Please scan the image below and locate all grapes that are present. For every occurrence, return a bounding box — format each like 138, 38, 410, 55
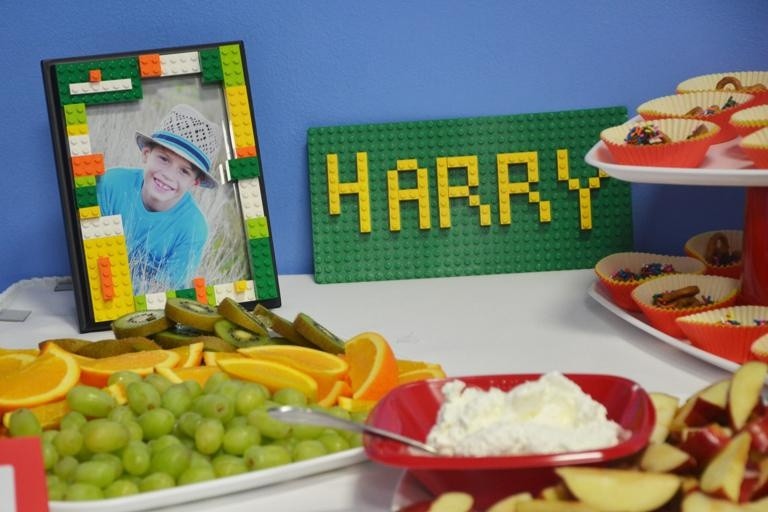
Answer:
8, 371, 368, 500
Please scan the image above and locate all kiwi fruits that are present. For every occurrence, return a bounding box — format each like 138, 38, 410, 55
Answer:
39, 296, 346, 359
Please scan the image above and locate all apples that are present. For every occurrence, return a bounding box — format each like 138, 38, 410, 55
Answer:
396, 360, 768, 512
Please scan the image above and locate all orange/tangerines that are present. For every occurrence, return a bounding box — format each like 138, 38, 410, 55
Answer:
0, 332, 446, 432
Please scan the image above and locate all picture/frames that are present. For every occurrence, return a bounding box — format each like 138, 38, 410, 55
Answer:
38, 37, 281, 332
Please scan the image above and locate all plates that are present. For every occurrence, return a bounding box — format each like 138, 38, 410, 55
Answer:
43, 448, 378, 511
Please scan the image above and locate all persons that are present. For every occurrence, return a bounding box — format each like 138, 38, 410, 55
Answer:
93, 99, 223, 297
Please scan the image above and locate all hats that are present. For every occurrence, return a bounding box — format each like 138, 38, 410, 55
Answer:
135, 104, 224, 191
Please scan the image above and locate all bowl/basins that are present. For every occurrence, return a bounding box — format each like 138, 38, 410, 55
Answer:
361, 376, 655, 502
